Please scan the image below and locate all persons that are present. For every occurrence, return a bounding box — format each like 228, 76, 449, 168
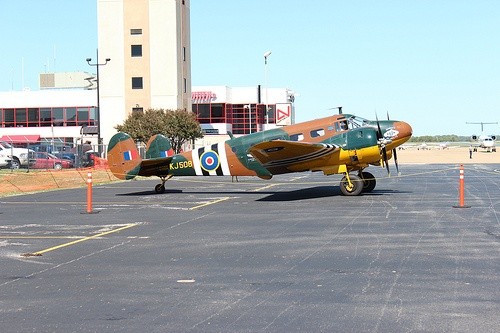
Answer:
468, 144, 473, 159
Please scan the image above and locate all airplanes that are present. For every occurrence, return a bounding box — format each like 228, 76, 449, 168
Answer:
466, 121, 500, 153
398, 142, 460, 152
106, 105, 412, 196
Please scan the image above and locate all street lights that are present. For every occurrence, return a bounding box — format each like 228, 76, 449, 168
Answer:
86, 48, 111, 157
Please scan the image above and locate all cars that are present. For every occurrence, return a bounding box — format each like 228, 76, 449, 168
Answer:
53, 152, 100, 168
29, 152, 72, 170
0, 146, 13, 169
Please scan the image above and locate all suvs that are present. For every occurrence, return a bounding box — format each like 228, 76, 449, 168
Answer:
0, 140, 36, 169
